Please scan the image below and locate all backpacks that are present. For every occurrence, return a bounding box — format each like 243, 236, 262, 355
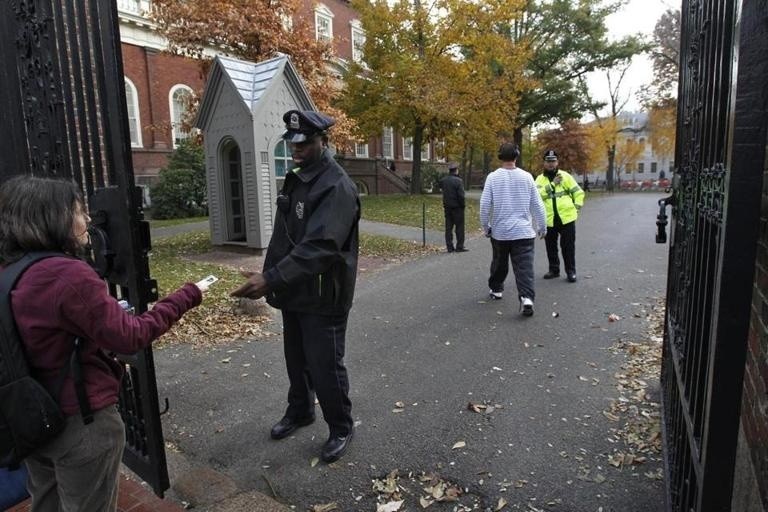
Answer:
0, 250, 94, 466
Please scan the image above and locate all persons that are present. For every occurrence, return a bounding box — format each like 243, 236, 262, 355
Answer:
227, 109, 362, 462
441, 160, 468, 253
534, 150, 586, 282
389, 159, 395, 175
478, 142, 548, 318
0, 173, 210, 511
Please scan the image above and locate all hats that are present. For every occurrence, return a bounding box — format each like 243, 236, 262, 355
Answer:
282, 110, 334, 143
543, 150, 558, 162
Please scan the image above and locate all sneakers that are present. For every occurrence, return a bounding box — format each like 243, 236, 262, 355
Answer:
489, 289, 502, 300
521, 296, 533, 316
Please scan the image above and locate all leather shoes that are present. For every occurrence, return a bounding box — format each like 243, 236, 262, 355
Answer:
271, 412, 316, 439
544, 272, 560, 278
322, 433, 352, 461
568, 272, 576, 282
456, 247, 469, 252
